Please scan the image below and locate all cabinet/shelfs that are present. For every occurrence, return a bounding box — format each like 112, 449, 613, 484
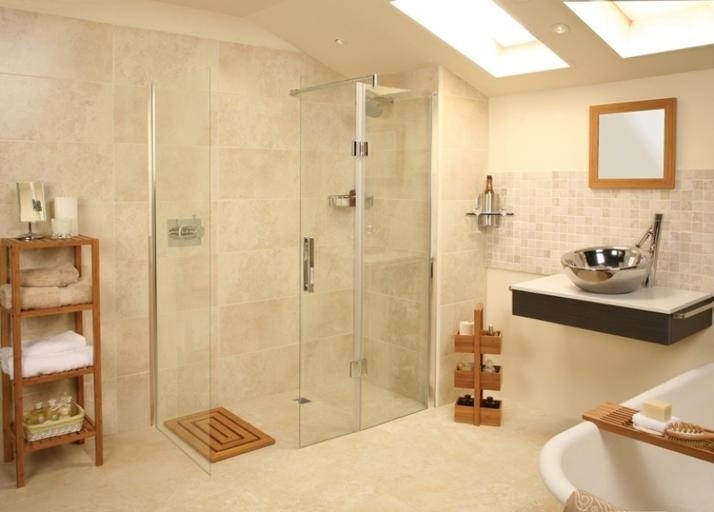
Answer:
2, 230, 104, 486
453, 304, 503, 428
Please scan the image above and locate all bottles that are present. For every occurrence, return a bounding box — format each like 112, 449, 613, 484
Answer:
29, 398, 60, 425
485, 175, 495, 194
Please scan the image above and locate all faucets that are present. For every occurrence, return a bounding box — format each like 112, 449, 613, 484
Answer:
635, 213, 663, 288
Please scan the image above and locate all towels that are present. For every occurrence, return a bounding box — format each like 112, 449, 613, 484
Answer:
2, 260, 95, 311
632, 413, 679, 437
1, 330, 94, 381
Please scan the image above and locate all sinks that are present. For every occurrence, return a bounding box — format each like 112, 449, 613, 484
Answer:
561, 246, 651, 294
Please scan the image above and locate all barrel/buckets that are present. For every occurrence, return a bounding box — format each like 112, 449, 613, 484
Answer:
479, 192, 501, 227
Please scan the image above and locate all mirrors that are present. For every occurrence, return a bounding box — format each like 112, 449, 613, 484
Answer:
587, 97, 678, 191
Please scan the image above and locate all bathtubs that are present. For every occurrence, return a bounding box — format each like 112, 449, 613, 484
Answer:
540, 362, 714, 512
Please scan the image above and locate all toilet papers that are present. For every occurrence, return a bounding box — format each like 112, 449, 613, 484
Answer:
460, 321, 474, 336
53, 196, 78, 235
